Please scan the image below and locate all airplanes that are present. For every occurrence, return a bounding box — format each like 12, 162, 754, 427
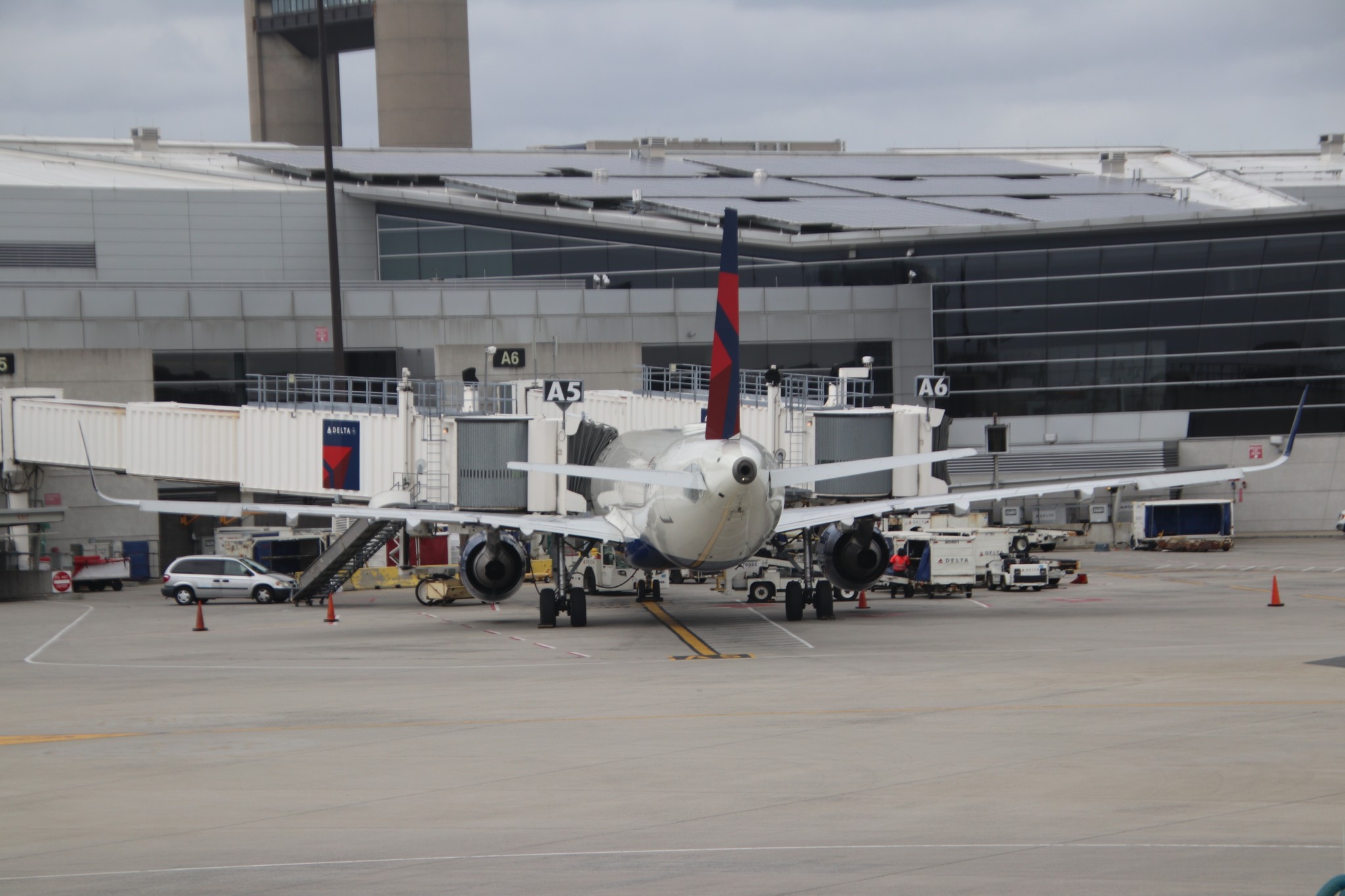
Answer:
94, 207, 1313, 628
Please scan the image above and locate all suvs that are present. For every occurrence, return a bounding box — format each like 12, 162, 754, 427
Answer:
161, 554, 300, 605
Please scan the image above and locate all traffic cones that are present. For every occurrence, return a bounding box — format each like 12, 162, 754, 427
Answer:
322, 593, 339, 622
190, 599, 209, 632
1266, 573, 1291, 610
856, 590, 874, 610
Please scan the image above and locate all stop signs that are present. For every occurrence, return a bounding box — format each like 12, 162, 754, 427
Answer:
51, 571, 74, 594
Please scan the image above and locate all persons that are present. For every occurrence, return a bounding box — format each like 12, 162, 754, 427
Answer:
887, 548, 914, 599
